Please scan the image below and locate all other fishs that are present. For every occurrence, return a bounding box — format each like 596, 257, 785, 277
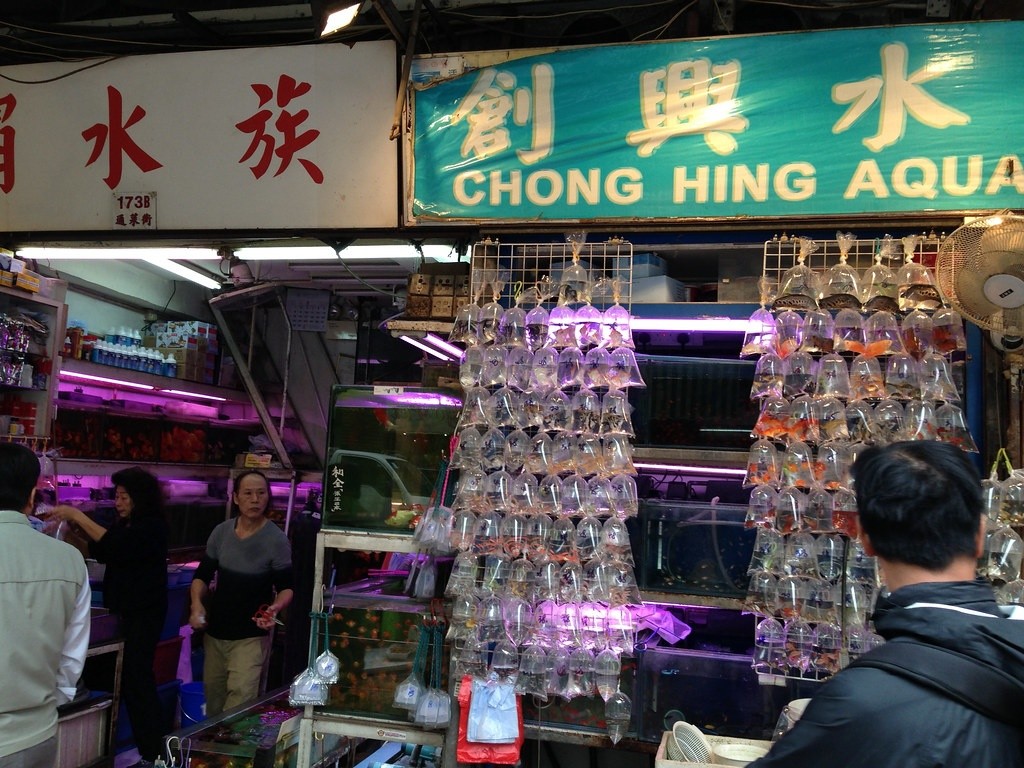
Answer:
190, 726, 255, 768
738, 264, 1024, 683
443, 299, 639, 704
318, 610, 450, 712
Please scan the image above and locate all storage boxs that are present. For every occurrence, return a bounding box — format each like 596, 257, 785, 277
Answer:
521, 655, 638, 729
655, 730, 773, 768
145, 319, 218, 383
208, 425, 251, 465
106, 410, 161, 462
161, 682, 300, 768
53, 401, 106, 460
320, 596, 449, 721
321, 382, 464, 534
645, 499, 756, 597
163, 415, 209, 464
58, 699, 113, 768
639, 646, 762, 743
0, 243, 67, 302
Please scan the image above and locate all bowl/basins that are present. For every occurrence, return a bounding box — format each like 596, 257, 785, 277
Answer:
710, 739, 771, 768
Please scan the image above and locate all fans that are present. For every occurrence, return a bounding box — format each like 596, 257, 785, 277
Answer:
935, 215, 1024, 353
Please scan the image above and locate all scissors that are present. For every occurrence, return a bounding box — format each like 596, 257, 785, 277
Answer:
255, 603, 285, 626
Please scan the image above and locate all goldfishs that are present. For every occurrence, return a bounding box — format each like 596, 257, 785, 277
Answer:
54, 419, 228, 463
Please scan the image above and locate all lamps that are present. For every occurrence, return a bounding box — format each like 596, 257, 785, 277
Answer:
15, 237, 455, 289
391, 329, 464, 362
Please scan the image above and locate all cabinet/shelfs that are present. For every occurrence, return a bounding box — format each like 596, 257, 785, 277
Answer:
97, 498, 225, 556
294, 443, 761, 768
0, 285, 70, 448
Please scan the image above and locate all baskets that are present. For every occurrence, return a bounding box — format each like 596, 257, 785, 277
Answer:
667, 720, 716, 764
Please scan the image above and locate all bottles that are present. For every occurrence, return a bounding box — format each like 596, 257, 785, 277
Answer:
105, 327, 142, 347
90, 338, 180, 378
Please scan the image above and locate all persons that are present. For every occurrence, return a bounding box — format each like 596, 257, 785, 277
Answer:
744, 439, 1024, 768
0, 441, 91, 768
46, 468, 167, 768
190, 472, 322, 719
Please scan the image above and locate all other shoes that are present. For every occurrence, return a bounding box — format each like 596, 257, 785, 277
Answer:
125, 759, 154, 768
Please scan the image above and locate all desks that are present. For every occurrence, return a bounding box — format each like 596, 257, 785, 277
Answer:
70, 639, 126, 768
